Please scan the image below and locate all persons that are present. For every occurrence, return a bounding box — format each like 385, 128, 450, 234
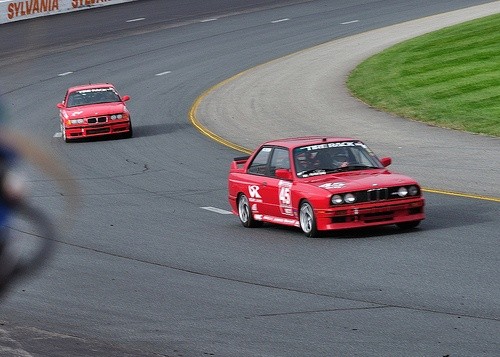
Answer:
329, 153, 349, 168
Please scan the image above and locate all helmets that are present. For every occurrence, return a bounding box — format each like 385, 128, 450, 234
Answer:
332, 147, 349, 166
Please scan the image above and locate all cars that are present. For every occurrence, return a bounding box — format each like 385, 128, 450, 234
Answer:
56, 82, 132, 141
226, 135, 425, 237
